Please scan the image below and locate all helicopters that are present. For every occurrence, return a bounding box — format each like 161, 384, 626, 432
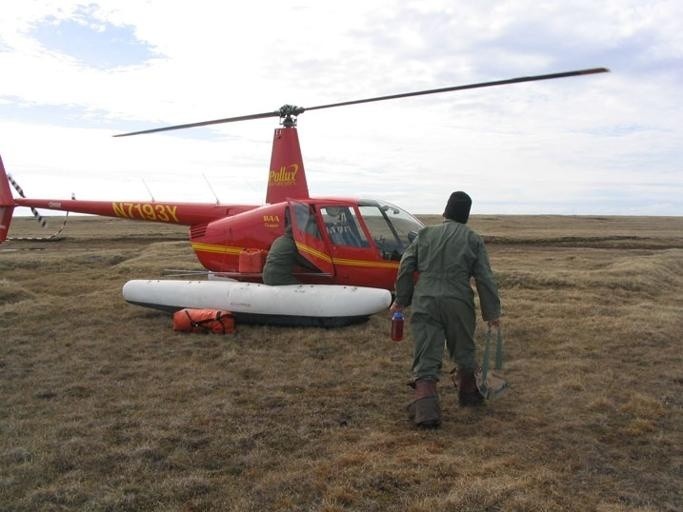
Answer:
0, 65, 610, 326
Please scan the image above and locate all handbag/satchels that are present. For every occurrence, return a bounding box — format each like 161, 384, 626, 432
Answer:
477, 368, 508, 399
173, 308, 234, 333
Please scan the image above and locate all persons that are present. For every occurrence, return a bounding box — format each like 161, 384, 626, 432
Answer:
263, 223, 322, 285
390, 192, 501, 426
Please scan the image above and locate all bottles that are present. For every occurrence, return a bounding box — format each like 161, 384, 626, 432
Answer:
390, 311, 403, 342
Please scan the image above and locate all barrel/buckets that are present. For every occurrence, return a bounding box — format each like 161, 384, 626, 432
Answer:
239, 247, 265, 274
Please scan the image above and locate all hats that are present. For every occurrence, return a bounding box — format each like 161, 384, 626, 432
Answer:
442, 191, 471, 223
